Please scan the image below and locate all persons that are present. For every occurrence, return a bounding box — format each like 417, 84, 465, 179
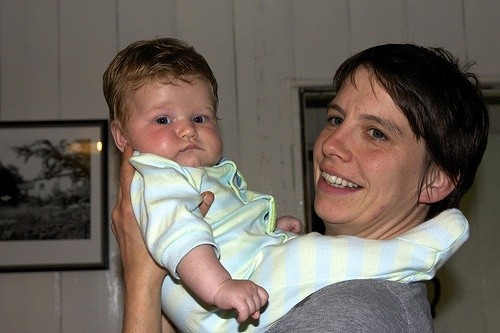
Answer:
117, 41, 491, 331
103, 31, 306, 333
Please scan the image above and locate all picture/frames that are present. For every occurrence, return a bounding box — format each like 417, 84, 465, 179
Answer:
1, 118, 109, 274
